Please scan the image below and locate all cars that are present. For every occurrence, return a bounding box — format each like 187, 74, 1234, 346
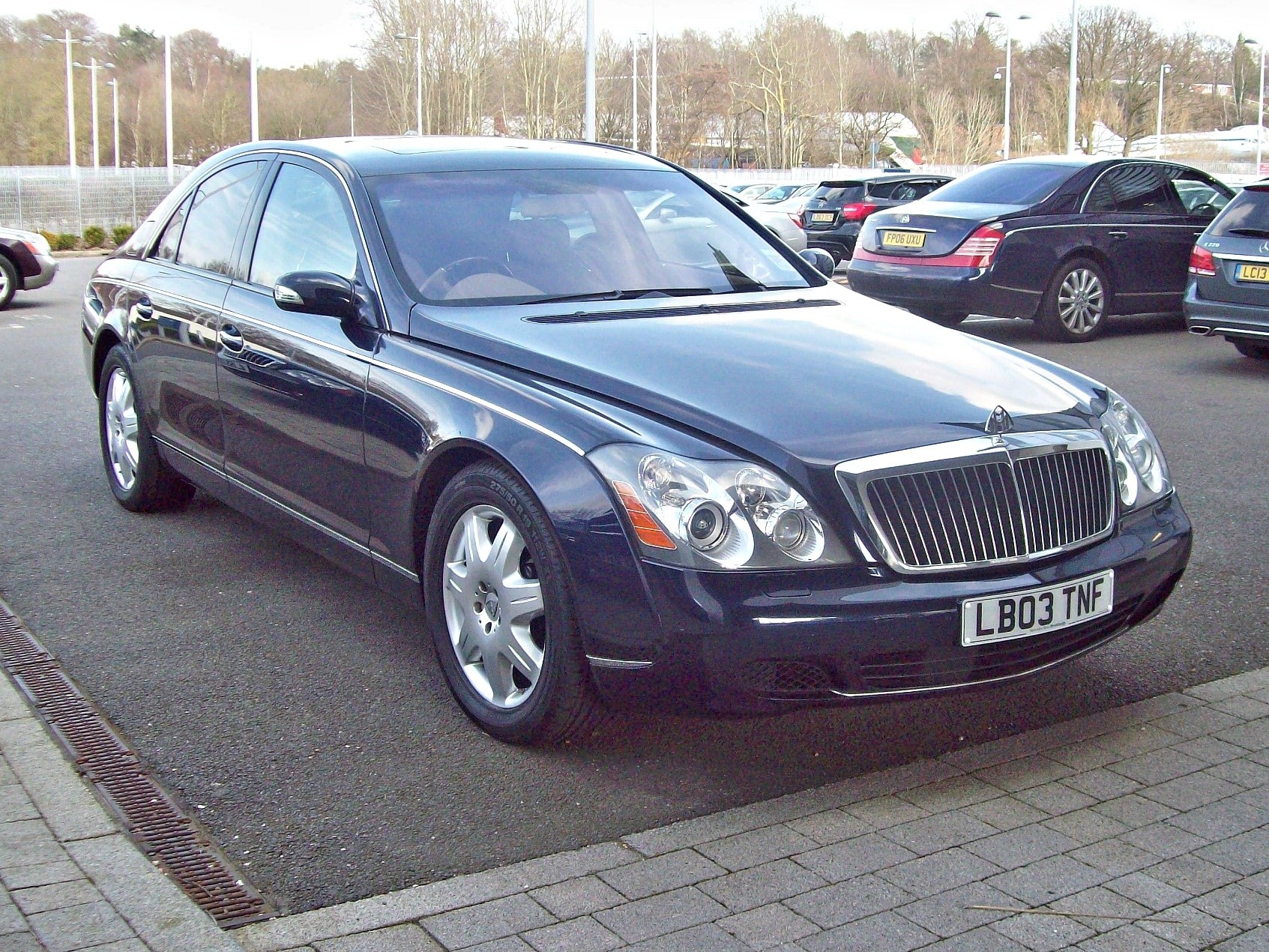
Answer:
80, 131, 1194, 750
570, 173, 819, 257
0, 226, 60, 311
845, 157, 1269, 360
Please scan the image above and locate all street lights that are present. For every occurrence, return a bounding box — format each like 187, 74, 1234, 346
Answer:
1156, 63, 1172, 158
629, 30, 649, 149
1243, 38, 1266, 182
394, 26, 423, 138
985, 9, 1032, 160
42, 28, 121, 179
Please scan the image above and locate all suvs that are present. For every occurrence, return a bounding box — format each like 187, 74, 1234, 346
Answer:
802, 169, 958, 266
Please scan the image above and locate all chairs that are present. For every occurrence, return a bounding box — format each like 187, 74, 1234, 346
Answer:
499, 219, 614, 295
912, 187, 930, 200
1140, 201, 1167, 213
